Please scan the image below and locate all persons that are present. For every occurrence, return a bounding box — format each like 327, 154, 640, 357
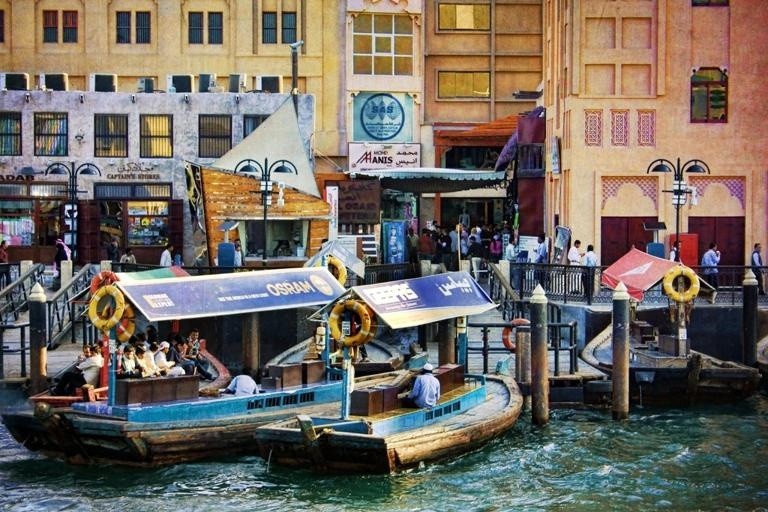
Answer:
568, 240, 585, 294
494, 223, 500, 235
429, 220, 439, 232
670, 240, 683, 265
581, 245, 598, 304
459, 207, 471, 229
479, 147, 500, 170
701, 242, 722, 292
107, 237, 122, 273
401, 363, 441, 408
234, 244, 243, 269
222, 369, 258, 398
490, 236, 502, 262
0, 239, 12, 288
501, 224, 510, 233
480, 224, 492, 261
464, 236, 483, 283
467, 228, 483, 245
450, 223, 463, 270
513, 203, 520, 246
407, 228, 419, 260
431, 232, 440, 263
159, 243, 175, 267
488, 223, 494, 232
461, 230, 470, 259
233, 237, 248, 267
120, 247, 137, 270
50, 325, 220, 395
54, 238, 74, 274
438, 228, 451, 270
751, 241, 766, 296
417, 229, 434, 260
405, 230, 412, 260
506, 236, 521, 289
533, 232, 549, 292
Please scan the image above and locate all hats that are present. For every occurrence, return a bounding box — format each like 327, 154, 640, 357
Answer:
423, 363, 434, 371
157, 341, 170, 350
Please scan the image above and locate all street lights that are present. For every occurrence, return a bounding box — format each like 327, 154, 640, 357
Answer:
647, 156, 709, 259
235, 156, 297, 260
45, 163, 101, 266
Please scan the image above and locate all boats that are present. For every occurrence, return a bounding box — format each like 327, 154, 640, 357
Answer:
254, 271, 522, 474
1, 266, 196, 454
581, 246, 762, 412
4, 271, 423, 463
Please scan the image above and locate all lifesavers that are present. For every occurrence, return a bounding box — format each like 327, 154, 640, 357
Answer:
314, 255, 348, 286
502, 319, 532, 354
102, 301, 136, 343
663, 265, 700, 303
330, 299, 372, 347
88, 284, 126, 329
337, 301, 377, 348
91, 271, 119, 296
115, 311, 128, 336
